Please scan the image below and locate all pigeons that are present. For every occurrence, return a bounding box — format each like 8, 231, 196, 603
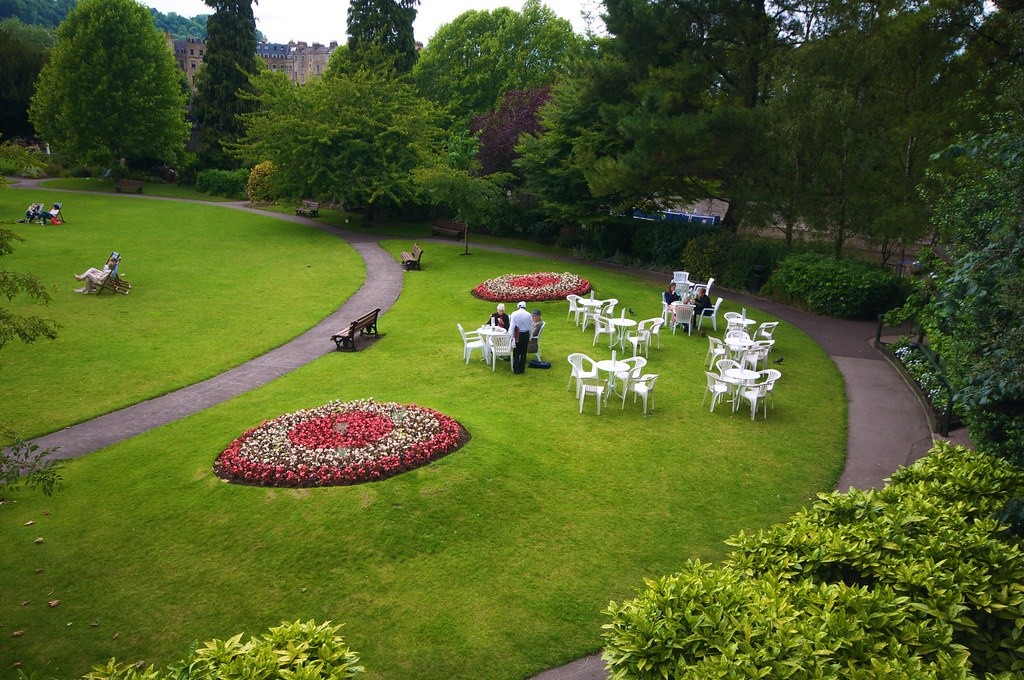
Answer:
773, 357, 783, 364
629, 308, 634, 314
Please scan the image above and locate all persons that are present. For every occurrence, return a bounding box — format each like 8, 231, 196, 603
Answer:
46, 143, 50, 155
508, 301, 533, 376
692, 287, 713, 328
26, 204, 40, 223
75, 257, 116, 295
665, 283, 681, 307
487, 303, 509, 331
504, 310, 542, 361
38, 204, 60, 226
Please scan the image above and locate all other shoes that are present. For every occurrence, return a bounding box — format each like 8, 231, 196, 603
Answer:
74, 273, 81, 282
82, 292, 90, 295
504, 357, 511, 361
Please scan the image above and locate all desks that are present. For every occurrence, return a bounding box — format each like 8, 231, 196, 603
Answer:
725, 369, 760, 412
729, 318, 757, 333
477, 326, 507, 362
724, 337, 754, 363
608, 318, 637, 355
595, 360, 630, 406
578, 299, 603, 329
674, 281, 695, 287
669, 301, 690, 331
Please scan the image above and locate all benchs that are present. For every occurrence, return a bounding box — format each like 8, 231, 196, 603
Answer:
431, 218, 465, 241
400, 243, 424, 271
115, 179, 145, 194
331, 308, 381, 352
296, 200, 319, 219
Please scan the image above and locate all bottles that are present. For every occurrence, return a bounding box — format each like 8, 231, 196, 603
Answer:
492, 323, 495, 333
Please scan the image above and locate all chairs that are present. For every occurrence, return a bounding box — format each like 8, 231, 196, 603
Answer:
25, 203, 44, 223
456, 271, 779, 421
49, 202, 66, 222
95, 251, 132, 295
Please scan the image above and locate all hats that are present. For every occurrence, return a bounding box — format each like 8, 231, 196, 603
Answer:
533, 310, 541, 315
517, 301, 526, 308
497, 304, 505, 310
